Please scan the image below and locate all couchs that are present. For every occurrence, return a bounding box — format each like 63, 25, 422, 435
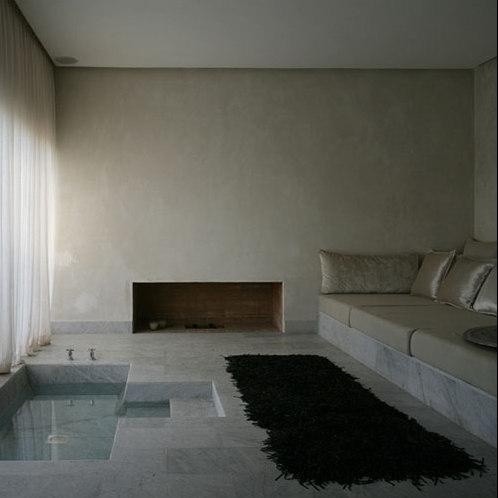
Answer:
316, 293, 497, 449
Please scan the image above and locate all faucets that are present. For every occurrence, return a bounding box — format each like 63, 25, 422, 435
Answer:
66, 349, 74, 360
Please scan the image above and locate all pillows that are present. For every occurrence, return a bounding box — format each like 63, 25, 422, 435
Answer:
319, 249, 419, 295
433, 254, 495, 312
471, 265, 497, 317
410, 249, 456, 303
462, 237, 497, 260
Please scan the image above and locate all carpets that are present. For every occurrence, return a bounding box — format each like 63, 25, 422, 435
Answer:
223, 354, 487, 493
